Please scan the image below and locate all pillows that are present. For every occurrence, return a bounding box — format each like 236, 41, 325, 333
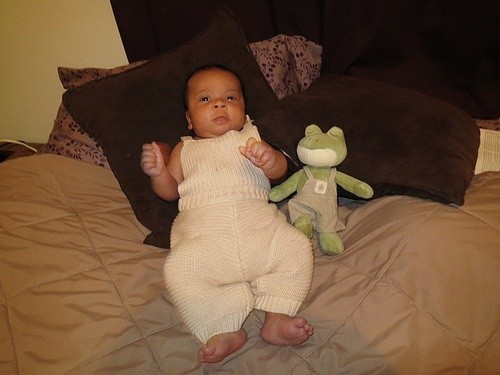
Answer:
30, 58, 148, 170
249, 35, 323, 99
62, 12, 284, 247
256, 67, 482, 205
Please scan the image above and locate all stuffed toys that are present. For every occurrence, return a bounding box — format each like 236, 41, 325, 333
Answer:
268, 125, 375, 256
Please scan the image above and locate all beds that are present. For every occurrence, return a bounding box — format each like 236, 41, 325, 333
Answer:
1, 142, 500, 375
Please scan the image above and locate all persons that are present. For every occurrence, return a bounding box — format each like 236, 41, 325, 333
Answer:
142, 64, 316, 364
